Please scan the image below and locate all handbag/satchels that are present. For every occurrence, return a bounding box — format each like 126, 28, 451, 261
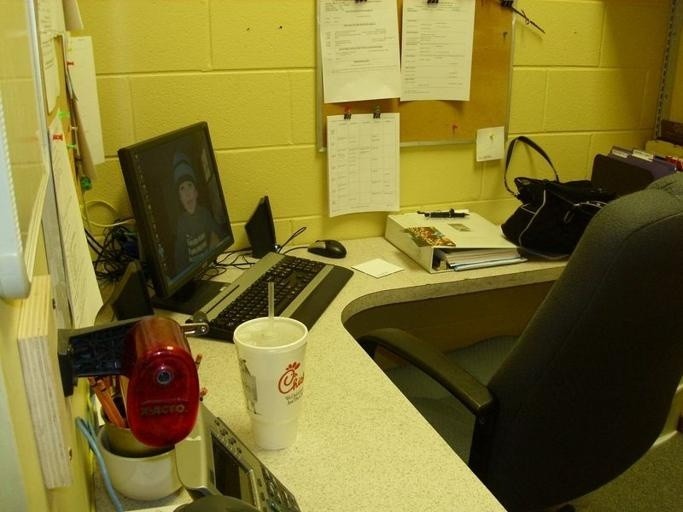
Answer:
501, 136, 623, 261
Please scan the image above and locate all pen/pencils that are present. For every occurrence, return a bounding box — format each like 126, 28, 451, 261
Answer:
90, 374, 127, 430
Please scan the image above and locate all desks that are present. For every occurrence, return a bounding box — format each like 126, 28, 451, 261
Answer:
92, 235, 569, 512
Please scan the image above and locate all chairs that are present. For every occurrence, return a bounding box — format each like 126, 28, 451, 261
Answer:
357, 172, 683, 512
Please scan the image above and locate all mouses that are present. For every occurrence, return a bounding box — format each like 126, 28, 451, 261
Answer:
307, 239, 346, 258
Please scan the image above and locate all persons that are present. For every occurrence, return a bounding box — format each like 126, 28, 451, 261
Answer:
172, 152, 222, 275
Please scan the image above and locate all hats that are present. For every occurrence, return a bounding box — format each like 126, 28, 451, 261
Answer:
172, 152, 198, 197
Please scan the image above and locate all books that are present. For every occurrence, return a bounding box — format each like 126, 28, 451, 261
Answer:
384, 209, 529, 274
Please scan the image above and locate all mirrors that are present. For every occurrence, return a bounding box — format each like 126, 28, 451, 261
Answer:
0, 0, 49, 299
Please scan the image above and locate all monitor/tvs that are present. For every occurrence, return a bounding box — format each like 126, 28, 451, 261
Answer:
116, 121, 233, 315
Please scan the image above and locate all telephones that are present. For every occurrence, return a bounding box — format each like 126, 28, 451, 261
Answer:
172, 396, 300, 512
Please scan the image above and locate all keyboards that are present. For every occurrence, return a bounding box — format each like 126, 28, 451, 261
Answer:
192, 251, 355, 345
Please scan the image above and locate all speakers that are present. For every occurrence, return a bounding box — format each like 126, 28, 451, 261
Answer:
244, 196, 277, 258
109, 261, 154, 321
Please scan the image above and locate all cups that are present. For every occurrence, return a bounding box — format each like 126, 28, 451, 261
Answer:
89, 428, 186, 501
100, 393, 170, 454
234, 315, 309, 451
